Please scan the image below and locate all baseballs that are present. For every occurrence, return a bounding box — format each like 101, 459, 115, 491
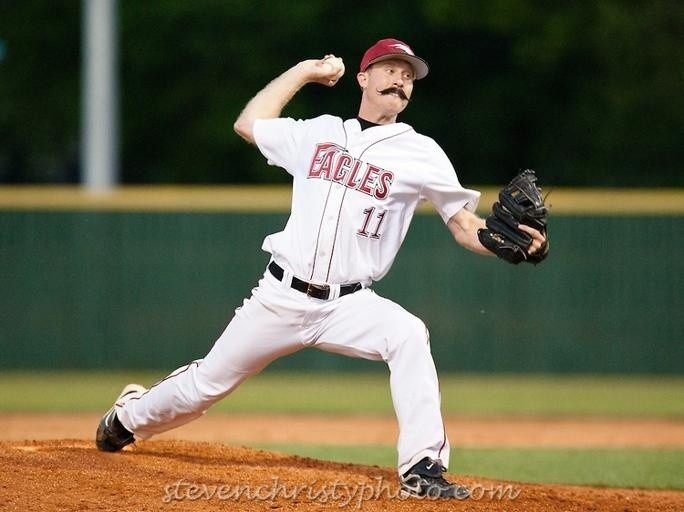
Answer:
323, 56, 344, 80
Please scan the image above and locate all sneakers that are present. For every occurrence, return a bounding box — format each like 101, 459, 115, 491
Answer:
97, 384, 147, 452
399, 460, 470, 501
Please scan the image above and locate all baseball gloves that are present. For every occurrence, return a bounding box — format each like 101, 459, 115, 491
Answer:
476, 168, 549, 265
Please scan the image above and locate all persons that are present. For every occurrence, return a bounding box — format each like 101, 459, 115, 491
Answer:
95, 35, 548, 501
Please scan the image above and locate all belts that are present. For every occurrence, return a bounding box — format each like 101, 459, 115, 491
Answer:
267, 261, 362, 300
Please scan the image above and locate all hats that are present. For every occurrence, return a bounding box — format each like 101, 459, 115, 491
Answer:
360, 38, 429, 81
